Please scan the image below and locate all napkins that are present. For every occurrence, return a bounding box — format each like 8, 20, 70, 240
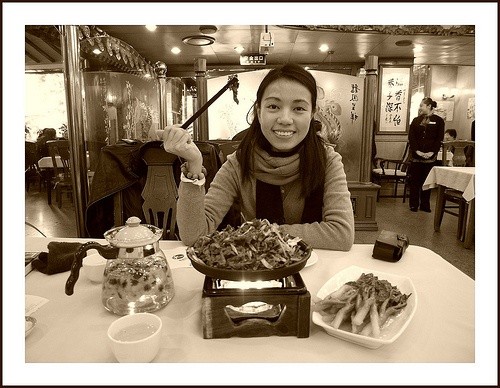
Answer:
31, 240, 87, 275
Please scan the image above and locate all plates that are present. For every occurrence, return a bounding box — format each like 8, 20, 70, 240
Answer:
185, 232, 312, 282
25, 316, 38, 339
312, 266, 418, 352
304, 251, 319, 268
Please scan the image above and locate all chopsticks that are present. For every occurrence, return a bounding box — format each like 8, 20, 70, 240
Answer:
180, 77, 237, 129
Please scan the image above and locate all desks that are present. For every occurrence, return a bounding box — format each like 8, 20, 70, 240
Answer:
38, 156, 72, 204
24, 235, 475, 363
422, 166, 475, 250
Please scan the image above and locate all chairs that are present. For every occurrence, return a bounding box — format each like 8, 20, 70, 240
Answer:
100, 141, 219, 242
440, 141, 475, 240
371, 141, 410, 203
25, 138, 75, 209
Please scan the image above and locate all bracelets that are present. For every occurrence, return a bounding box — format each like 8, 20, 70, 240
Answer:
180, 161, 208, 181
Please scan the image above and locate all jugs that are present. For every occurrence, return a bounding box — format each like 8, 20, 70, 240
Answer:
65, 216, 176, 317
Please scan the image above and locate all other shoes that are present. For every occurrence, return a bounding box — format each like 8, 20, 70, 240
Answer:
420, 207, 431, 212
410, 206, 418, 212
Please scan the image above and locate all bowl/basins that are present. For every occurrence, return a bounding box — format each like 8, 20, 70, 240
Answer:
80, 252, 108, 283
106, 312, 163, 363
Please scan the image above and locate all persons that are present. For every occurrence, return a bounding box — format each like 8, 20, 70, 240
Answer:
445, 128, 457, 140
408, 96, 445, 212
155, 63, 354, 252
